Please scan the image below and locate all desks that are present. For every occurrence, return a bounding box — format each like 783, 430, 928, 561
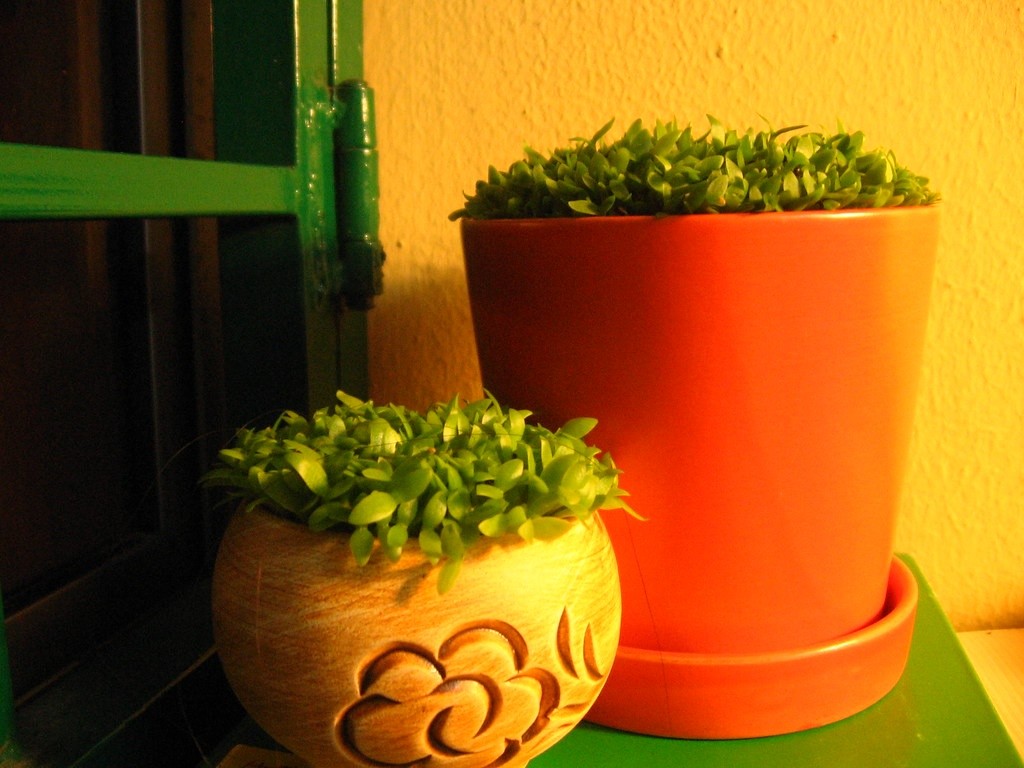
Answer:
195, 552, 1024, 768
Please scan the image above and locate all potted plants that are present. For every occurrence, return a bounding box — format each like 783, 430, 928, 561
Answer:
447, 113, 944, 740
218, 389, 646, 768
